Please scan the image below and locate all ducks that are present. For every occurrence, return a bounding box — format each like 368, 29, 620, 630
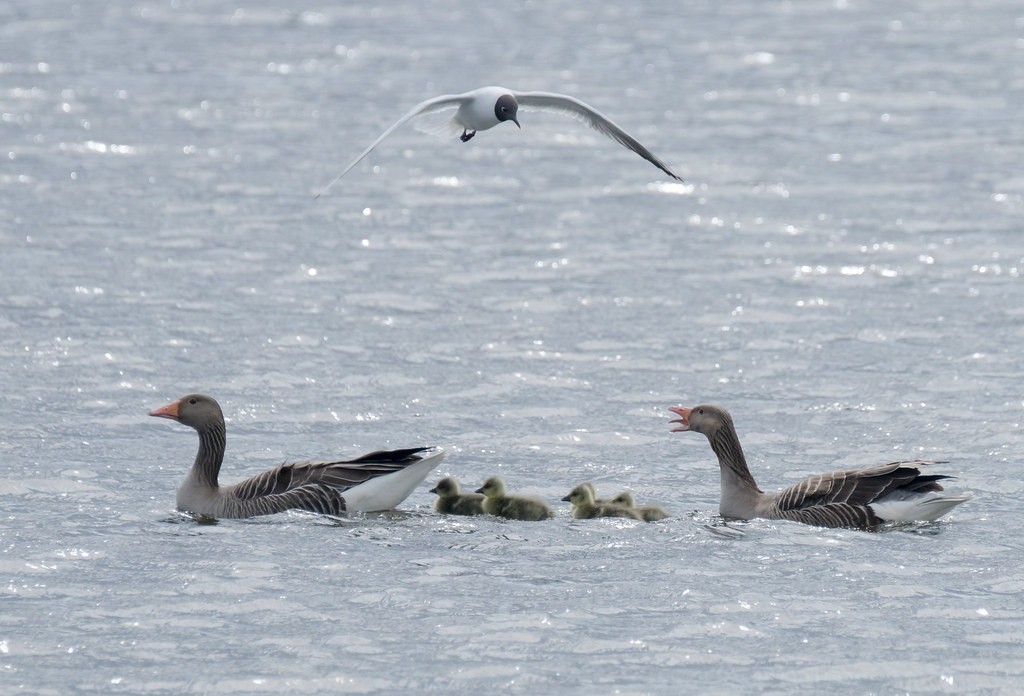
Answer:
668, 405, 969, 534
428, 478, 485, 516
561, 483, 672, 523
148, 394, 448, 520
475, 476, 557, 521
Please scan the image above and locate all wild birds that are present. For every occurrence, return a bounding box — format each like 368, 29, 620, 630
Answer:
314, 86, 685, 198
